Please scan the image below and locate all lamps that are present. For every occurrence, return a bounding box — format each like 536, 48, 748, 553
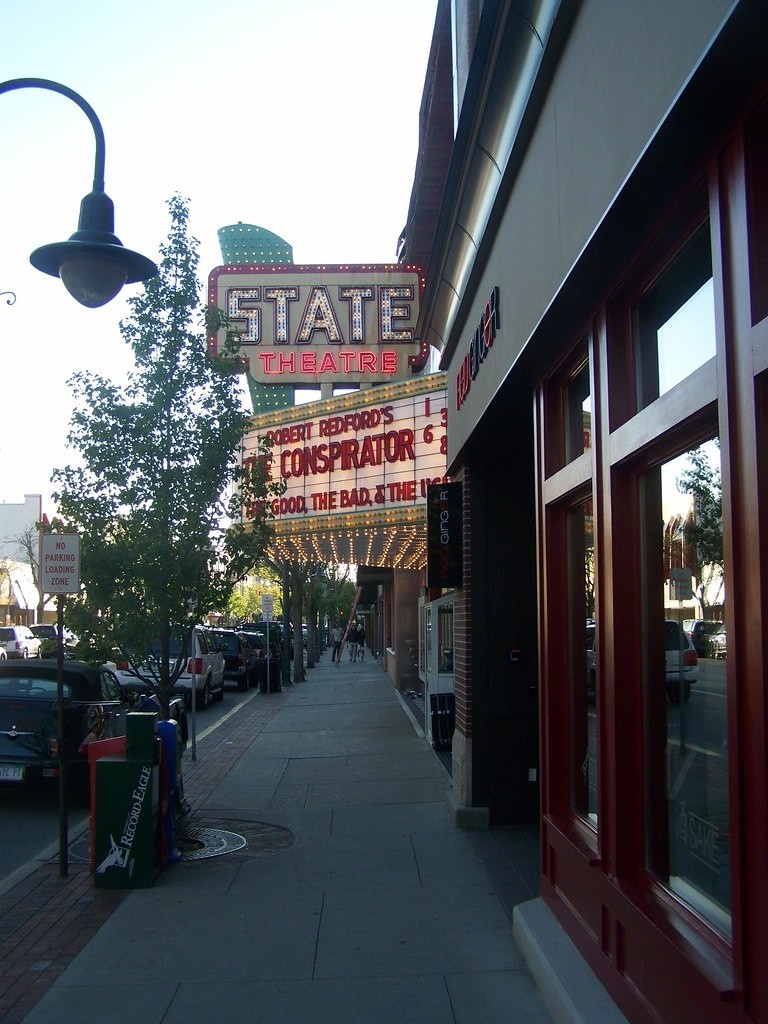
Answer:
1, 76, 159, 312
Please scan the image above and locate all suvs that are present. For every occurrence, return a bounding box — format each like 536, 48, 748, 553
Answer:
95, 622, 225, 711
580, 618, 699, 703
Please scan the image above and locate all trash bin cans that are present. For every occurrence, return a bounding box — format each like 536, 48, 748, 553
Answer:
258, 655, 283, 694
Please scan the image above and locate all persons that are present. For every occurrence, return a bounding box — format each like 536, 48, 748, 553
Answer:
357, 624, 365, 663
330, 623, 345, 662
343, 624, 360, 662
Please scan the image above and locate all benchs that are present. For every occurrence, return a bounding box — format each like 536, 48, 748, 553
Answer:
0, 687, 67, 701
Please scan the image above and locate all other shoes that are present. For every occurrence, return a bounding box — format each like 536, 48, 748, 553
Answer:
353, 660, 356, 663
331, 660, 335, 663
350, 659, 352, 662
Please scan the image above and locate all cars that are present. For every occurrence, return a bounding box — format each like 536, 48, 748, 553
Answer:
28, 623, 72, 660
0, 658, 143, 811
682, 619, 728, 659
202, 619, 330, 693
1, 622, 43, 660
